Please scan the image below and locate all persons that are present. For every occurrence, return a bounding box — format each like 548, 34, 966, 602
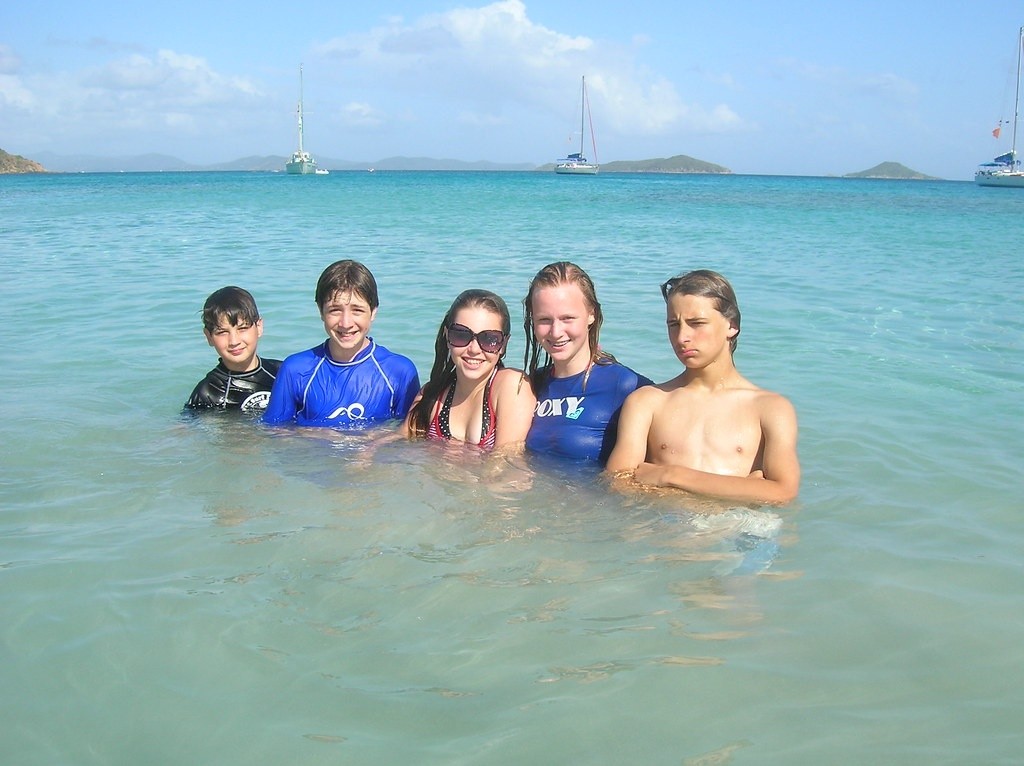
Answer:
606, 269, 800, 515
181, 286, 284, 421
518, 262, 654, 480
404, 290, 538, 455
260, 259, 421, 434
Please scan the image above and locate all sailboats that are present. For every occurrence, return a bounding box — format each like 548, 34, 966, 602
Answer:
556, 76, 599, 175
974, 26, 1023, 187
286, 62, 318, 178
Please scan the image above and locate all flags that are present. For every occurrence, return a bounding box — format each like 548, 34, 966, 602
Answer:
993, 127, 1000, 138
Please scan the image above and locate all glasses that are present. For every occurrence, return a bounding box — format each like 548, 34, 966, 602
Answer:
447, 322, 506, 354
